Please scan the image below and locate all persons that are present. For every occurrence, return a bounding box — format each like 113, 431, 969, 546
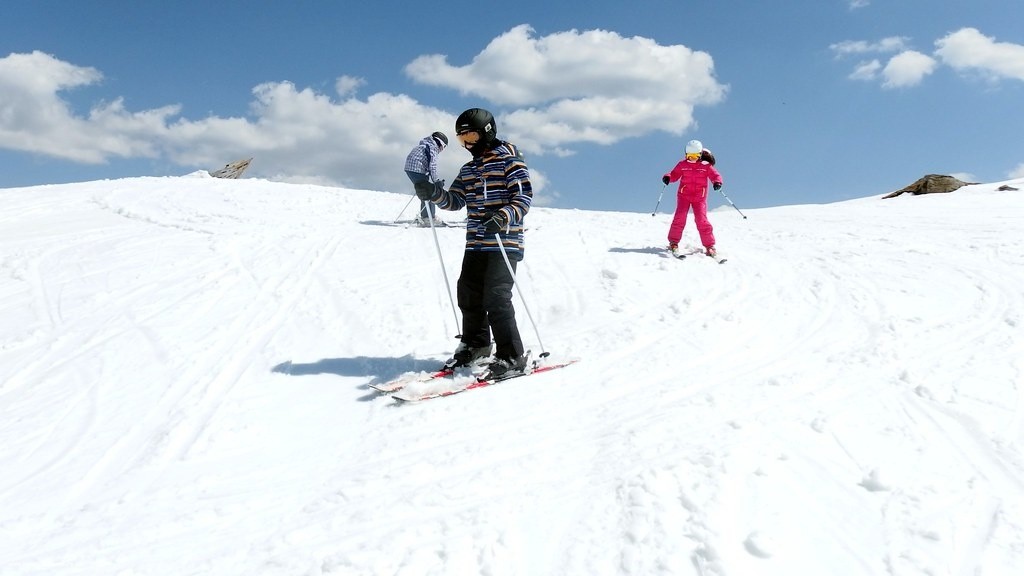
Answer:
404, 132, 448, 226
415, 108, 532, 377
663, 140, 723, 254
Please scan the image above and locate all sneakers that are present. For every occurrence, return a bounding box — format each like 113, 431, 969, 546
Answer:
477, 352, 528, 385
423, 216, 445, 228
440, 341, 493, 374
706, 246, 716, 256
669, 242, 681, 253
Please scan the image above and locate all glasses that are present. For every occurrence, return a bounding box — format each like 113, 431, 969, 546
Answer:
456, 129, 483, 147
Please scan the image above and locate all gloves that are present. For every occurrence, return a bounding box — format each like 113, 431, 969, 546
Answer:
713, 182, 721, 191
662, 176, 670, 186
416, 181, 440, 201
480, 213, 506, 239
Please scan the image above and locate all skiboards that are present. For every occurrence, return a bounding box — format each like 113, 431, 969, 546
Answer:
394, 218, 469, 228
366, 356, 581, 405
668, 239, 728, 266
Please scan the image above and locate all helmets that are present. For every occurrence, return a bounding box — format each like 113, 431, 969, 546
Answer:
455, 108, 497, 152
685, 139, 703, 160
431, 131, 448, 150
